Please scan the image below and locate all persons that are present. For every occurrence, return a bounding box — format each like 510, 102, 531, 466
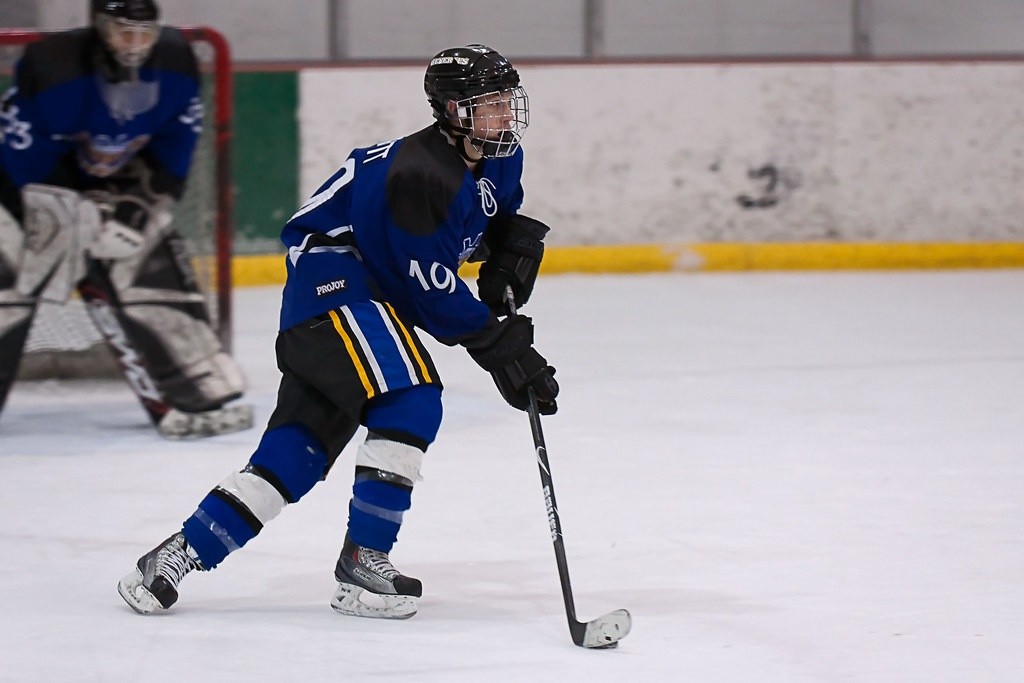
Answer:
0, 0, 206, 416
115, 44, 559, 619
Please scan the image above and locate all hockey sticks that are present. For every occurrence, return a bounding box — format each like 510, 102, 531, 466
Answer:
63, 250, 255, 438
500, 285, 632, 647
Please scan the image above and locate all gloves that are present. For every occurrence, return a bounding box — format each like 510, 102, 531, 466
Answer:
467, 213, 551, 318
464, 314, 559, 415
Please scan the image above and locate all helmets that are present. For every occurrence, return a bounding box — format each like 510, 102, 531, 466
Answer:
424, 43, 530, 159
89, 0, 160, 69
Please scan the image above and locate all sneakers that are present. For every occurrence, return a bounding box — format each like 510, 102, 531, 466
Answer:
331, 534, 422, 620
116, 531, 211, 615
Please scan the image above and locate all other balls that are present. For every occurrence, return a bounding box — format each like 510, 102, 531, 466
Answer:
593, 642, 619, 650
